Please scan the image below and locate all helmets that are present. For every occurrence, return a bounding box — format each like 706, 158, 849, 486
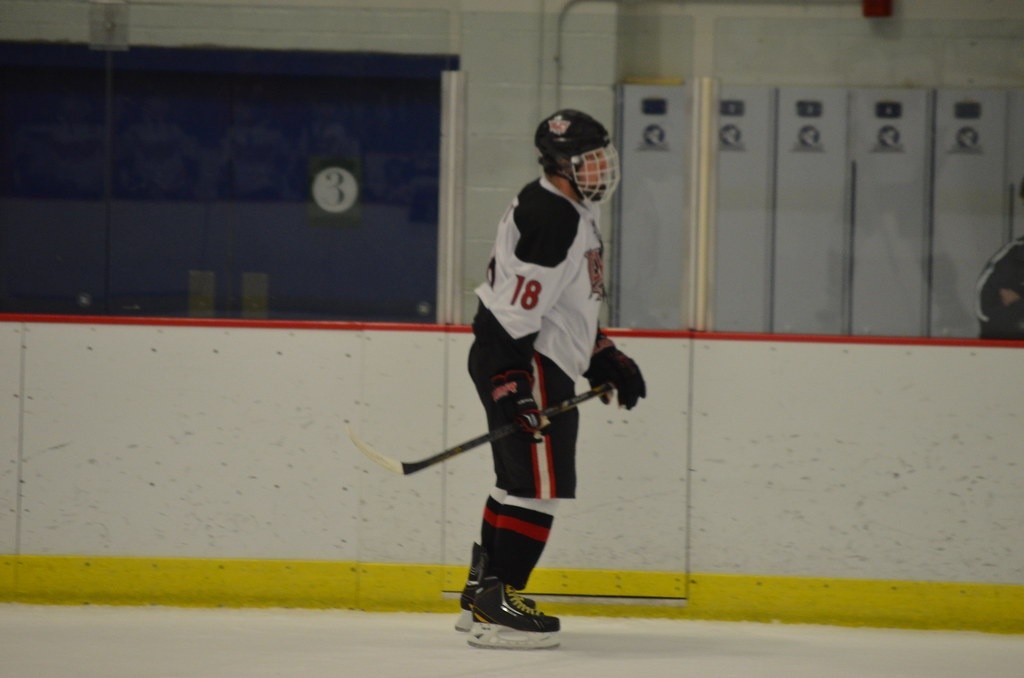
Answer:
534, 109, 620, 205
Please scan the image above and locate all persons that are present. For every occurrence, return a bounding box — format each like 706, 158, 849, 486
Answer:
972, 235, 1024, 341
455, 109, 649, 647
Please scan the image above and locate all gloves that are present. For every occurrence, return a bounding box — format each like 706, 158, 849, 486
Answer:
582, 334, 646, 410
489, 369, 552, 443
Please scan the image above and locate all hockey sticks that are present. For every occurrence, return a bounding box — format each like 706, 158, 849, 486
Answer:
347, 380, 615, 477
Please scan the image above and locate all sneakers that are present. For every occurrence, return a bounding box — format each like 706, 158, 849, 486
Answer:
455, 541, 537, 632
467, 553, 562, 647
